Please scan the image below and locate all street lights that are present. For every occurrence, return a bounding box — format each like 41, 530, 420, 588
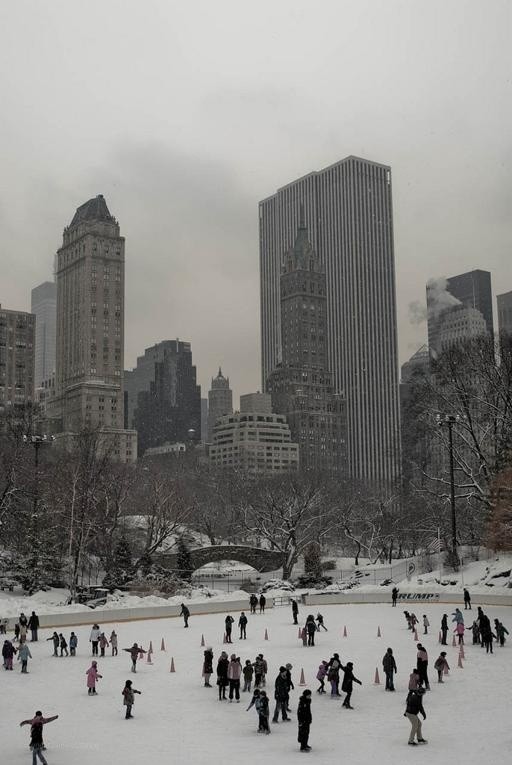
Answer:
434, 412, 469, 574
22, 433, 56, 562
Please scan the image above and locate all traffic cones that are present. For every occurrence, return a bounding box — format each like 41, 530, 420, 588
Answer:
300, 667, 307, 687
298, 627, 302, 638
200, 634, 205, 646
140, 637, 165, 662
374, 667, 382, 685
439, 631, 465, 676
223, 632, 227, 643
343, 625, 347, 637
414, 628, 419, 642
377, 626, 381, 637
264, 628, 268, 639
169, 657, 176, 673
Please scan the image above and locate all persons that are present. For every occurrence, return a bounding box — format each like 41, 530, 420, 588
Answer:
301, 613, 328, 646
27, 721, 48, 764
86, 661, 103, 695
0, 611, 39, 674
403, 607, 509, 653
259, 594, 266, 612
246, 663, 313, 752
89, 623, 146, 673
179, 603, 191, 627
249, 593, 258, 614
47, 631, 78, 657
316, 653, 363, 710
224, 612, 248, 644
392, 587, 399, 607
463, 587, 471, 608
202, 646, 267, 703
383, 643, 450, 745
122, 679, 141, 719
290, 598, 299, 624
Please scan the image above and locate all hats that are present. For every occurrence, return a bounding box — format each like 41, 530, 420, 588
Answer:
279, 666, 286, 672
303, 690, 311, 695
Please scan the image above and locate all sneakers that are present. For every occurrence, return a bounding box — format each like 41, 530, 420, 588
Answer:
418, 739, 426, 742
300, 745, 311, 750
266, 730, 271, 734
408, 741, 417, 745
273, 719, 278, 722
346, 704, 353, 708
257, 729, 265, 733
283, 718, 290, 721
